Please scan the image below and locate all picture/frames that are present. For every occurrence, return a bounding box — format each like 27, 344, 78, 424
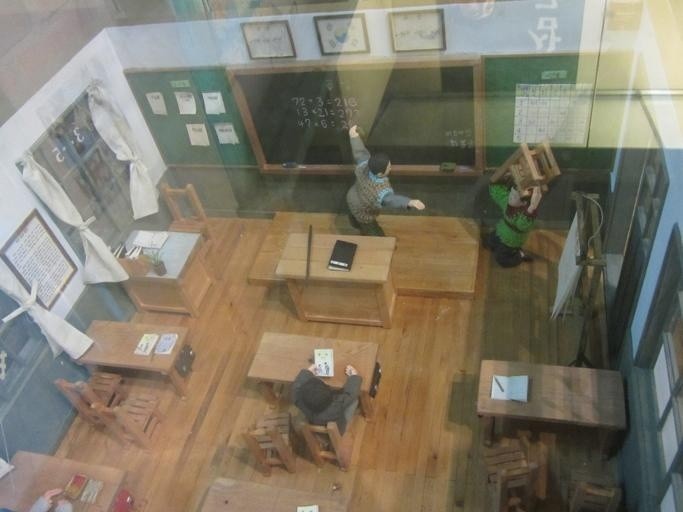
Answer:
240, 19, 297, 62
311, 15, 371, 57
386, 8, 447, 53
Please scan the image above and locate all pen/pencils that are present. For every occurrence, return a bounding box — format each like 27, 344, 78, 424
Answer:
494, 376, 505, 393
151, 252, 161, 266
282, 161, 298, 168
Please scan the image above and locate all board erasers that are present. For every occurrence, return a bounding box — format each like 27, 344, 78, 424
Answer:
440, 162, 457, 172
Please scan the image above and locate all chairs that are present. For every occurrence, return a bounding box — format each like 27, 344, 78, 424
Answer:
158, 180, 218, 263
488, 138, 562, 197
479, 437, 623, 512
239, 410, 355, 472
54, 371, 165, 449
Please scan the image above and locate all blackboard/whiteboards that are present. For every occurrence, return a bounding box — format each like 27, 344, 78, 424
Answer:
483, 56, 580, 174
124, 64, 256, 167
224, 54, 482, 176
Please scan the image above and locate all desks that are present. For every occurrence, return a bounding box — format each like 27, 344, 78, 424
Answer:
72, 319, 200, 398
199, 476, 350, 512
474, 359, 629, 462
245, 332, 383, 424
114, 227, 213, 319
0, 450, 124, 512
